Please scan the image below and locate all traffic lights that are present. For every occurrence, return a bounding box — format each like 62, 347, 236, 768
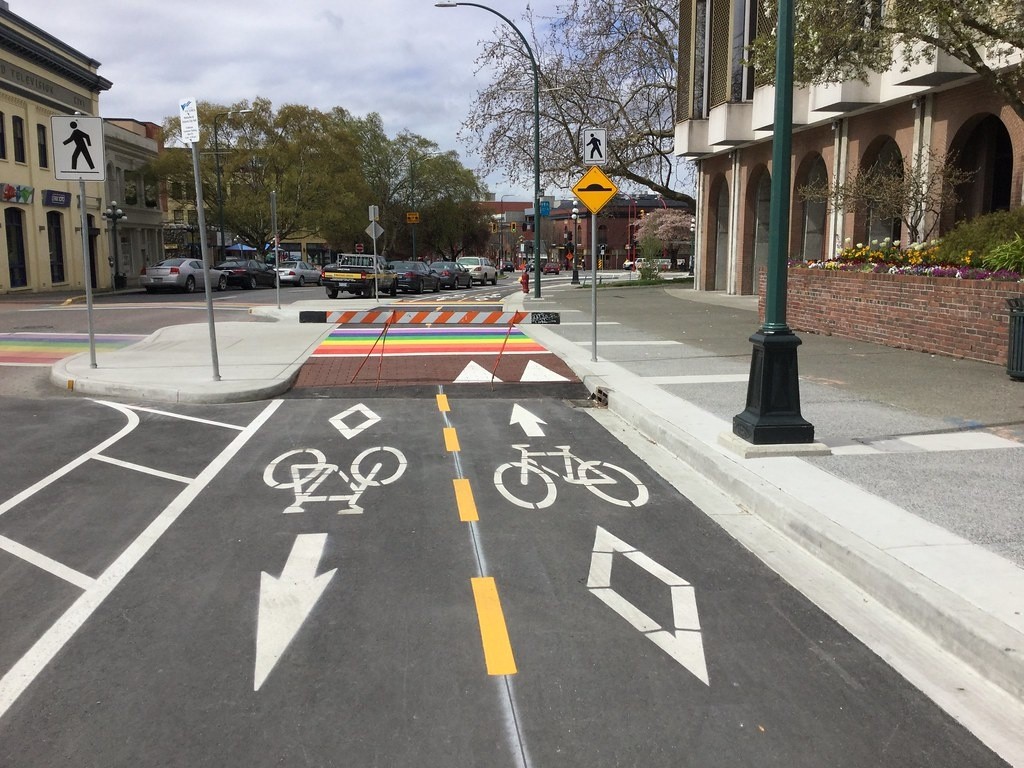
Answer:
492, 222, 497, 234
510, 221, 516, 233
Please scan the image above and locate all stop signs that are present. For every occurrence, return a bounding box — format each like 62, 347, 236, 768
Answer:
355, 244, 363, 252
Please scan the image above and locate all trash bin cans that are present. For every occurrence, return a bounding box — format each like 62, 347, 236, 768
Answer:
1005, 296, 1024, 381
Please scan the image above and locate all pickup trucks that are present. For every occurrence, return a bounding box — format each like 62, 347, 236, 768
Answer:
322, 253, 399, 298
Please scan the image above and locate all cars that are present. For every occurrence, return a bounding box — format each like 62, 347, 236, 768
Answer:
213, 260, 280, 289
502, 261, 515, 273
273, 260, 324, 287
139, 258, 227, 294
456, 256, 497, 285
530, 260, 545, 272
389, 261, 441, 293
430, 262, 473, 289
543, 263, 559, 275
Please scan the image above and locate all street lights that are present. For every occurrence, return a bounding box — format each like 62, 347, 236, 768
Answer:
571, 200, 581, 285
501, 194, 520, 260
689, 218, 695, 276
434, 1, 542, 298
101, 201, 128, 289
